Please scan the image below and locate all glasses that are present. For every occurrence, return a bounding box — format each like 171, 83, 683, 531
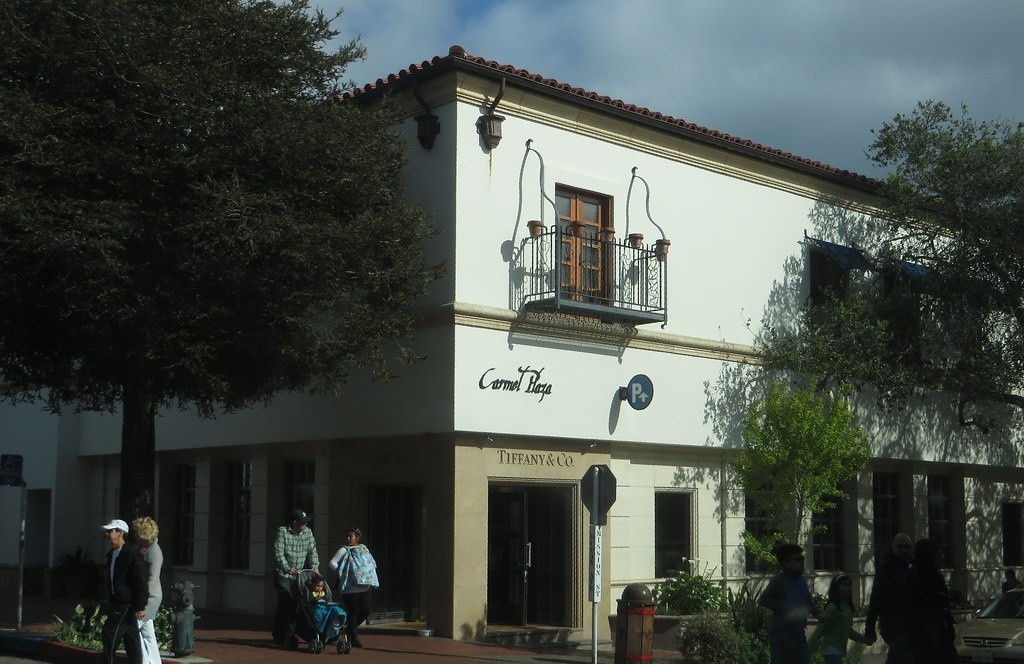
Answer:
935, 552, 945, 558
786, 556, 806, 562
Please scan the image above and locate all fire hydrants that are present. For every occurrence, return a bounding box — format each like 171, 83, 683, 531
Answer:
165, 579, 199, 658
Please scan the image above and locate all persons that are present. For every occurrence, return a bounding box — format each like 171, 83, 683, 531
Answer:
1002, 569, 1024, 593
807, 574, 877, 664
864, 532, 915, 664
330, 528, 378, 648
131, 514, 164, 664
758, 544, 825, 664
914, 539, 970, 664
100, 519, 149, 664
272, 510, 320, 646
309, 575, 326, 603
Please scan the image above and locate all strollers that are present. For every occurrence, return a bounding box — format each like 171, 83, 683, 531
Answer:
283, 569, 352, 656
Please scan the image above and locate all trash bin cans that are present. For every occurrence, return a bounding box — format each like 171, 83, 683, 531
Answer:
615, 598, 655, 664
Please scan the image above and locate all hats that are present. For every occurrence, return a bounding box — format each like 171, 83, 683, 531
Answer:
101, 519, 129, 534
294, 510, 312, 522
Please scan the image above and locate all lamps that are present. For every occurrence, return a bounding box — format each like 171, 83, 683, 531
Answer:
961, 413, 998, 434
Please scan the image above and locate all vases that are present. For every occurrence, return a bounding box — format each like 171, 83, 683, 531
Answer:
602, 226, 615, 241
656, 238, 671, 254
527, 220, 543, 237
629, 233, 643, 248
572, 220, 586, 235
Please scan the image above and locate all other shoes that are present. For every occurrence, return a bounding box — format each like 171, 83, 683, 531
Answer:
347, 633, 363, 647
273, 636, 285, 646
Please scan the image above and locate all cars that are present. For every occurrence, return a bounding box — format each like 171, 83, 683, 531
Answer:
953, 588, 1024, 663
